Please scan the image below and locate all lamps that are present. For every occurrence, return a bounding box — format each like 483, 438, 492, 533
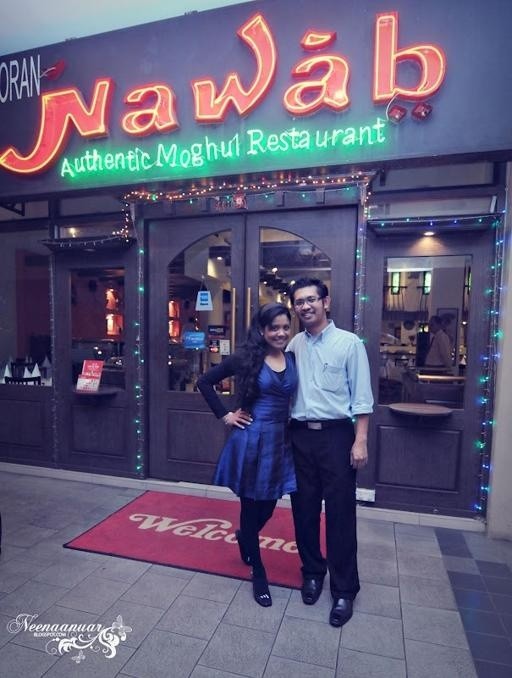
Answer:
169, 300, 181, 338
103, 287, 123, 335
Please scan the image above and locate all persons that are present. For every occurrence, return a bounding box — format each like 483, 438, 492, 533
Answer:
195, 300, 301, 609
420, 313, 455, 373
279, 275, 375, 630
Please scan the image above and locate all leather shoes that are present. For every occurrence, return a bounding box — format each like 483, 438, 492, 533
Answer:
235, 527, 254, 565
329, 596, 354, 627
250, 568, 272, 607
300, 560, 328, 605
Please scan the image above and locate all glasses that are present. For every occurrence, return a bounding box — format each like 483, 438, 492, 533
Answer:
292, 295, 325, 309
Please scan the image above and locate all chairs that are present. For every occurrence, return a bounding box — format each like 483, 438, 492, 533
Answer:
5, 338, 190, 393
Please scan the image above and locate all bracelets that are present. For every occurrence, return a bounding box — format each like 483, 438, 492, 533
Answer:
224, 411, 232, 426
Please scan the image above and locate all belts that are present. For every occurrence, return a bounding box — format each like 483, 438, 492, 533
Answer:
290, 417, 350, 430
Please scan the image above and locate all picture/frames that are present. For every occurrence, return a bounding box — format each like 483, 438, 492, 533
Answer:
436, 307, 457, 367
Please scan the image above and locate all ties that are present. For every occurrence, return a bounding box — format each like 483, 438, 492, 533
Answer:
430, 335, 435, 347
